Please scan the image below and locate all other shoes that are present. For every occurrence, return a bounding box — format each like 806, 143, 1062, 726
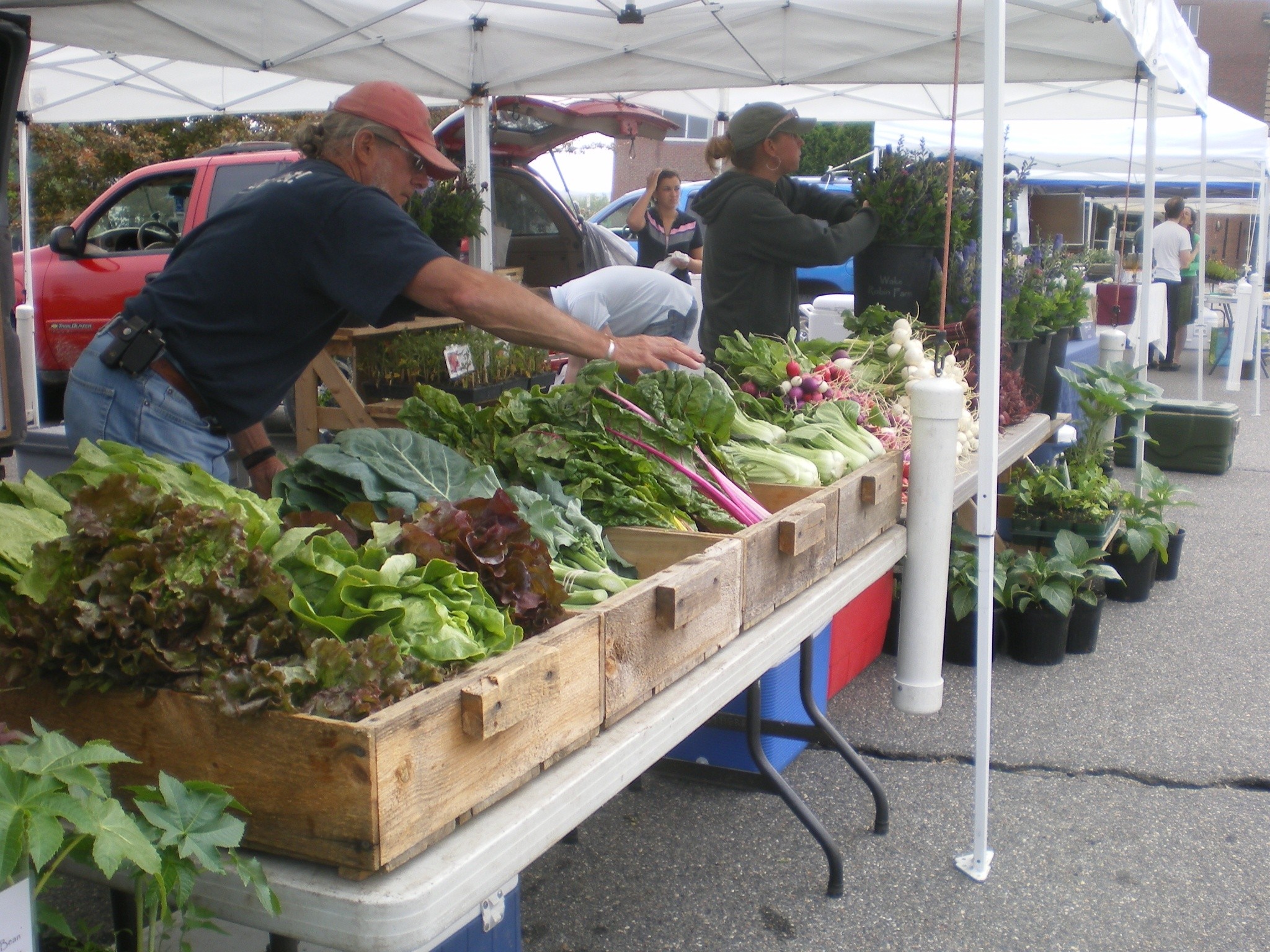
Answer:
1147, 360, 1159, 369
1158, 361, 1179, 371
1172, 362, 1182, 368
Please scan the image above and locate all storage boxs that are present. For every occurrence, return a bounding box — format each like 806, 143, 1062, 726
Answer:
828, 567, 896, 701
1096, 281, 1137, 327
1185, 304, 1220, 350
1115, 396, 1243, 475
756, 427, 905, 562
295, 870, 523, 952
614, 477, 839, 630
561, 523, 744, 734
0, 609, 604, 875
663, 615, 833, 771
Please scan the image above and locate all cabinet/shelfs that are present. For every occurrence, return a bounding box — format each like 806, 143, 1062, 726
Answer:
293, 316, 490, 458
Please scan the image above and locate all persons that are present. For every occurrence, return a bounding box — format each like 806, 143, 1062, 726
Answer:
528, 264, 698, 386
690, 101, 880, 368
1133, 195, 1200, 371
626, 168, 704, 285
64, 81, 706, 499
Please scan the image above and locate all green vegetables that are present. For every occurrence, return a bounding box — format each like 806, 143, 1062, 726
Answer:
0, 359, 886, 738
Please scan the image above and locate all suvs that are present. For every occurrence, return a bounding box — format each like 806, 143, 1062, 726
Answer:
10, 91, 681, 437
580, 162, 855, 303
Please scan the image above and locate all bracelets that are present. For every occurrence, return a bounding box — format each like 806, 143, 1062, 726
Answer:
243, 445, 275, 470
605, 333, 615, 362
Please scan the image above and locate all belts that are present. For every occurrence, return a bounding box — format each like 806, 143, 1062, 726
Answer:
108, 321, 207, 420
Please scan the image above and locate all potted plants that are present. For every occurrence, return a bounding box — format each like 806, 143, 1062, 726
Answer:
846, 121, 1203, 669
343, 159, 558, 406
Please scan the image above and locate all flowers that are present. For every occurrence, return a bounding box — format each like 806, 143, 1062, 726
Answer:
1003, 230, 1068, 331
1051, 231, 1103, 328
924, 236, 1027, 342
896, 121, 1039, 247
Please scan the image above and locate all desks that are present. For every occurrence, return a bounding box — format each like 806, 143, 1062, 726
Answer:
1079, 283, 1170, 377
997, 335, 1101, 495
1206, 293, 1270, 380
0, 401, 1074, 952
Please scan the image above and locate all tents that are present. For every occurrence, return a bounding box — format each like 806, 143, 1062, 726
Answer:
0, 0, 1210, 882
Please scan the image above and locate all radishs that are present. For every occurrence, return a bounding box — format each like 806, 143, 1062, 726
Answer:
781, 381, 792, 393
791, 375, 803, 387
818, 381, 830, 393
887, 318, 979, 464
833, 358, 858, 369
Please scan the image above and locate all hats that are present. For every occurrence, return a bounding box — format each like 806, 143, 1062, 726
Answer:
726, 101, 818, 154
335, 81, 461, 181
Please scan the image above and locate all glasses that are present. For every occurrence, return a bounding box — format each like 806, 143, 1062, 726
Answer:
764, 107, 799, 141
347, 131, 425, 172
